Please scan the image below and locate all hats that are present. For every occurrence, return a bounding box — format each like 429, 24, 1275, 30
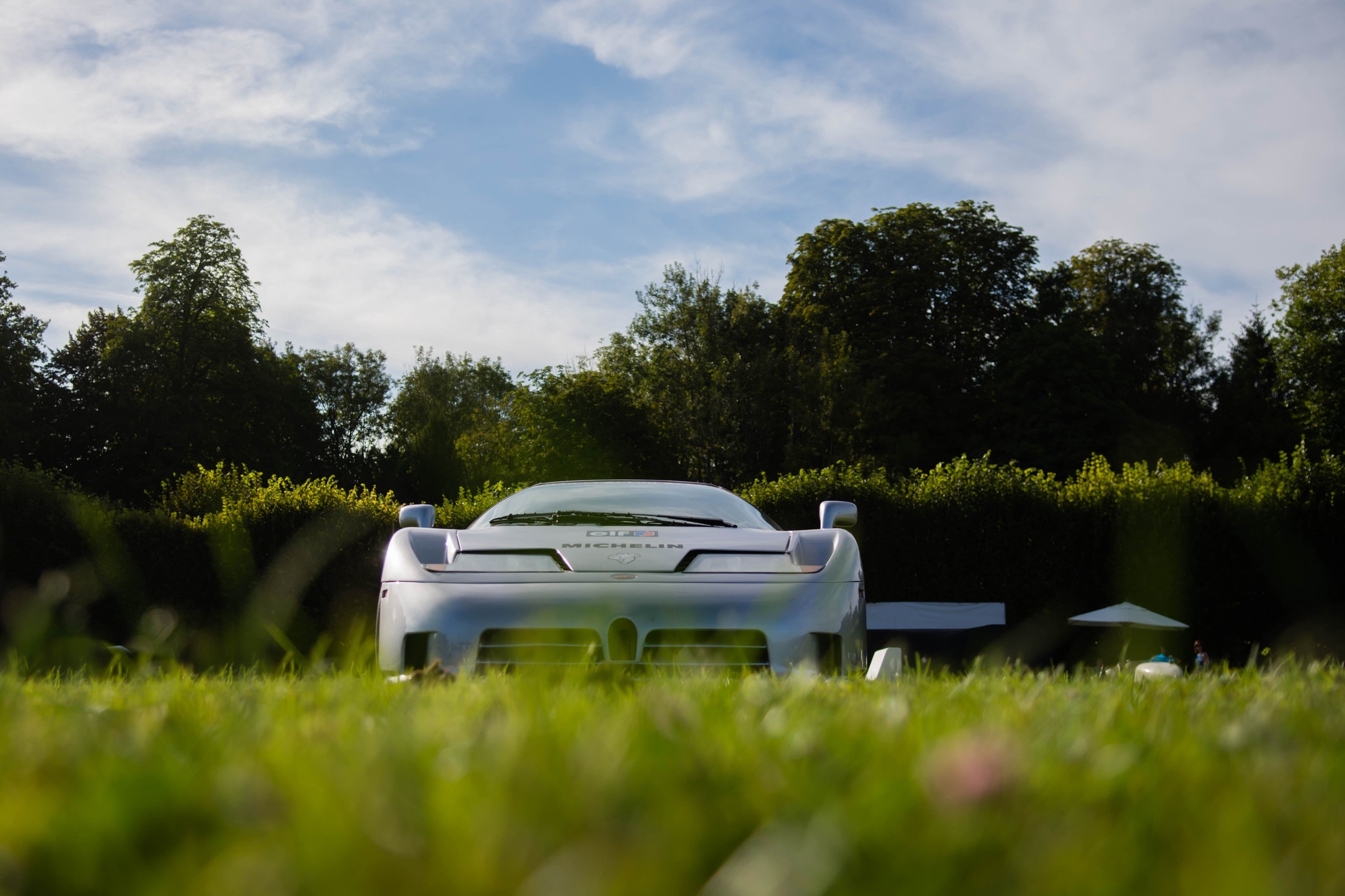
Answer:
1194, 640, 1201, 646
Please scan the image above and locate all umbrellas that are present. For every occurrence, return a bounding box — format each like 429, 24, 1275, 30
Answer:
1068, 601, 1190, 668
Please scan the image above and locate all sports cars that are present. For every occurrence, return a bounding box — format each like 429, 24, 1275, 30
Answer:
375, 475, 870, 679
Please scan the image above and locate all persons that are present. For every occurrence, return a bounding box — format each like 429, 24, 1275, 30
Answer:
1194, 640, 1209, 674
1150, 645, 1177, 664
